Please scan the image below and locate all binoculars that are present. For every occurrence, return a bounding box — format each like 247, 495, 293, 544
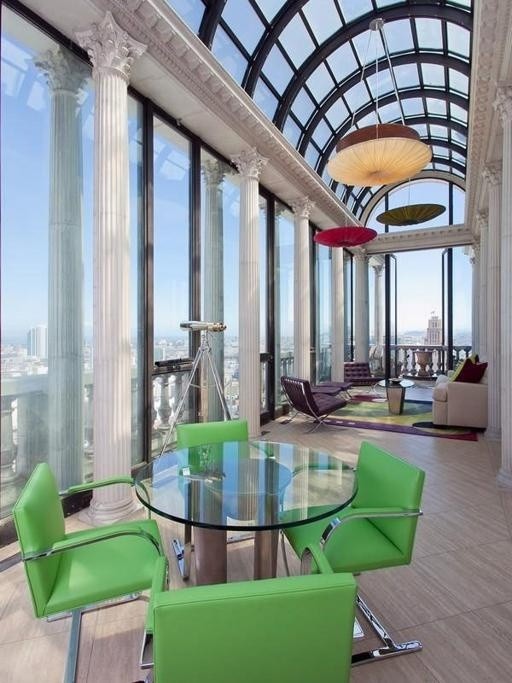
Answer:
179, 321, 227, 332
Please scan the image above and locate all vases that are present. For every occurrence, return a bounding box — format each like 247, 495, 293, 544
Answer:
413, 351, 432, 376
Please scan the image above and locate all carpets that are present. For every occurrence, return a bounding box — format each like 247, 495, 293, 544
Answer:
313, 394, 478, 441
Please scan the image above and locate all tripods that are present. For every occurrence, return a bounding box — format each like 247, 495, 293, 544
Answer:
154, 346, 232, 469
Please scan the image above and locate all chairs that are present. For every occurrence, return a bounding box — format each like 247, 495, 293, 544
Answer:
171, 421, 279, 578
12, 462, 164, 683
276, 441, 428, 670
280, 361, 384, 432
143, 543, 358, 681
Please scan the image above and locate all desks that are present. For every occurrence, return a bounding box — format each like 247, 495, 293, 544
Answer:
132, 440, 357, 669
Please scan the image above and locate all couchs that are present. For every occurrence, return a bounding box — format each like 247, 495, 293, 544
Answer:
432, 370, 488, 428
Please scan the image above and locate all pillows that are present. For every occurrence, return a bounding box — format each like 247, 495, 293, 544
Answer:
449, 353, 488, 383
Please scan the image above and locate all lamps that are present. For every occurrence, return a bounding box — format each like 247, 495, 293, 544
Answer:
313, 185, 377, 247
375, 177, 446, 226
326, 18, 432, 187
